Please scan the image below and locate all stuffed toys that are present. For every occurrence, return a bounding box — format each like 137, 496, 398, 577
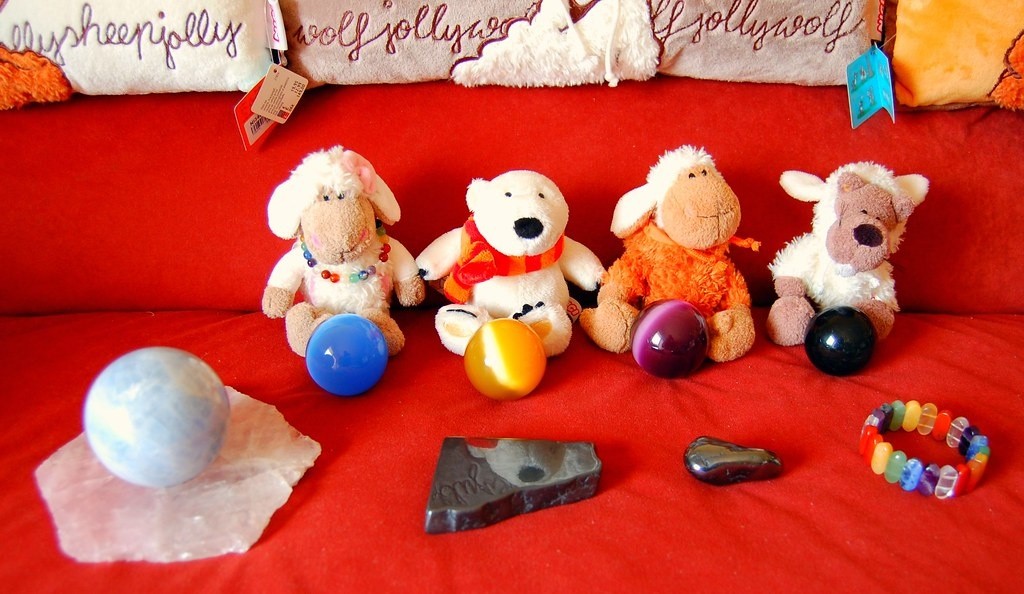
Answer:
416, 171, 606, 359
261, 145, 426, 358
767, 162, 930, 346
580, 145, 756, 364
464, 436, 598, 488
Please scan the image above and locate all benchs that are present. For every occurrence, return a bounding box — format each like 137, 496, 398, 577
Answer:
0, 75, 1024, 594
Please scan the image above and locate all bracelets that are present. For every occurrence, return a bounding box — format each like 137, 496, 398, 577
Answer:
858, 400, 990, 500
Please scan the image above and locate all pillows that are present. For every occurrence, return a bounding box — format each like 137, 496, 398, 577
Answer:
0, 0, 287, 112
278, 0, 884, 87
883, 0, 1024, 113
